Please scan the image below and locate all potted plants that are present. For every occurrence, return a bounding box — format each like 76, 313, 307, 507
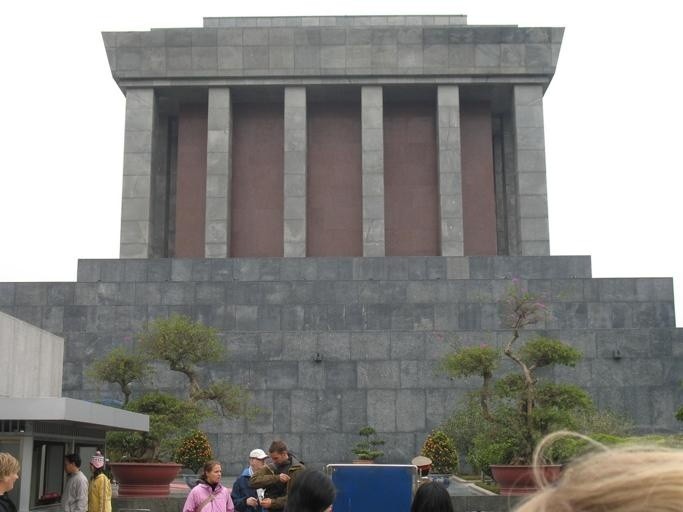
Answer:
432, 278, 595, 497
82, 312, 273, 497
175, 430, 212, 490
351, 426, 388, 464
420, 429, 458, 491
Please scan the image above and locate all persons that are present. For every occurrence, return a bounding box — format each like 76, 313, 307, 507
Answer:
285, 471, 335, 511
88, 449, 111, 511
229, 447, 275, 510
0, 452, 20, 511
60, 452, 88, 511
412, 482, 452, 512
410, 454, 433, 487
180, 458, 235, 512
246, 440, 306, 509
508, 431, 683, 511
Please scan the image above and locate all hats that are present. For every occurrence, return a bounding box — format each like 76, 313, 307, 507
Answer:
88, 449, 104, 468
247, 447, 269, 460
410, 455, 432, 470
